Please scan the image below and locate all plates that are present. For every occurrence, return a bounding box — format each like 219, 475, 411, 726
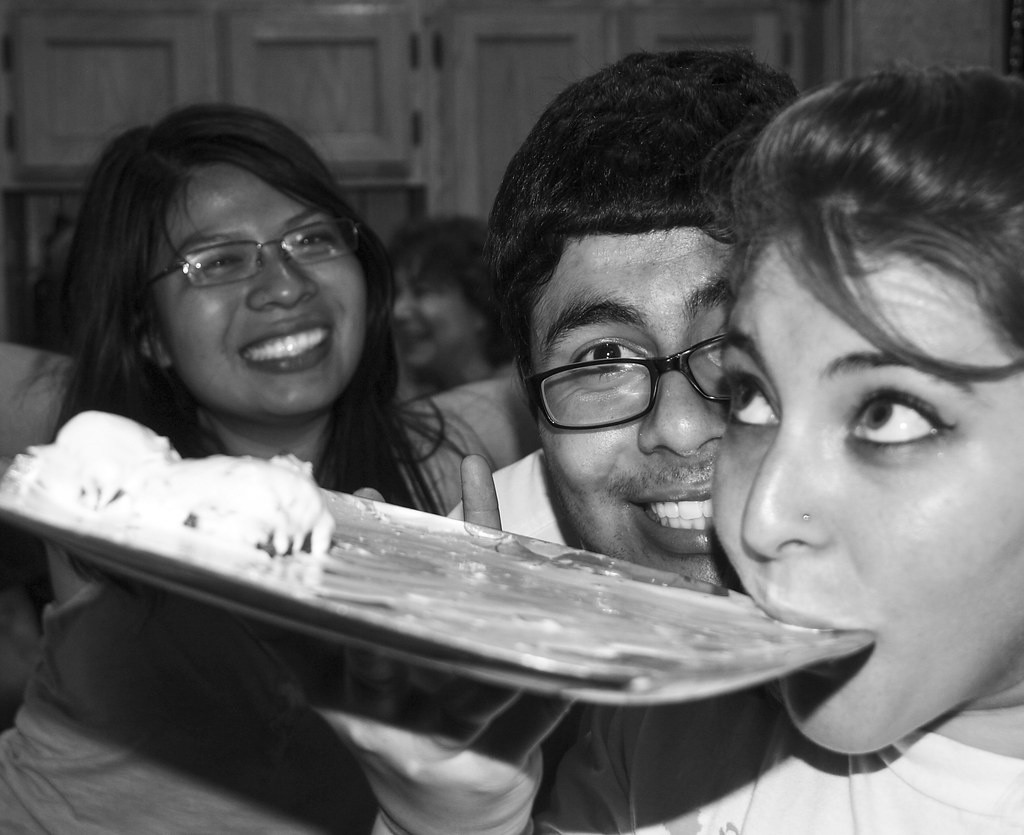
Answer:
0, 478, 876, 706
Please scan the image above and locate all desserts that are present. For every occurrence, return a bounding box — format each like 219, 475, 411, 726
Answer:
0, 411, 337, 581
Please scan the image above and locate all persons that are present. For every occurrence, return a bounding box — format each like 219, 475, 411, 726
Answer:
436, 47, 797, 594
264, 61, 1024, 835
388, 216, 514, 387
0, 106, 540, 835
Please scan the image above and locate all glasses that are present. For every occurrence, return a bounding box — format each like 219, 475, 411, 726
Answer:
524, 330, 731, 431
153, 218, 361, 288
